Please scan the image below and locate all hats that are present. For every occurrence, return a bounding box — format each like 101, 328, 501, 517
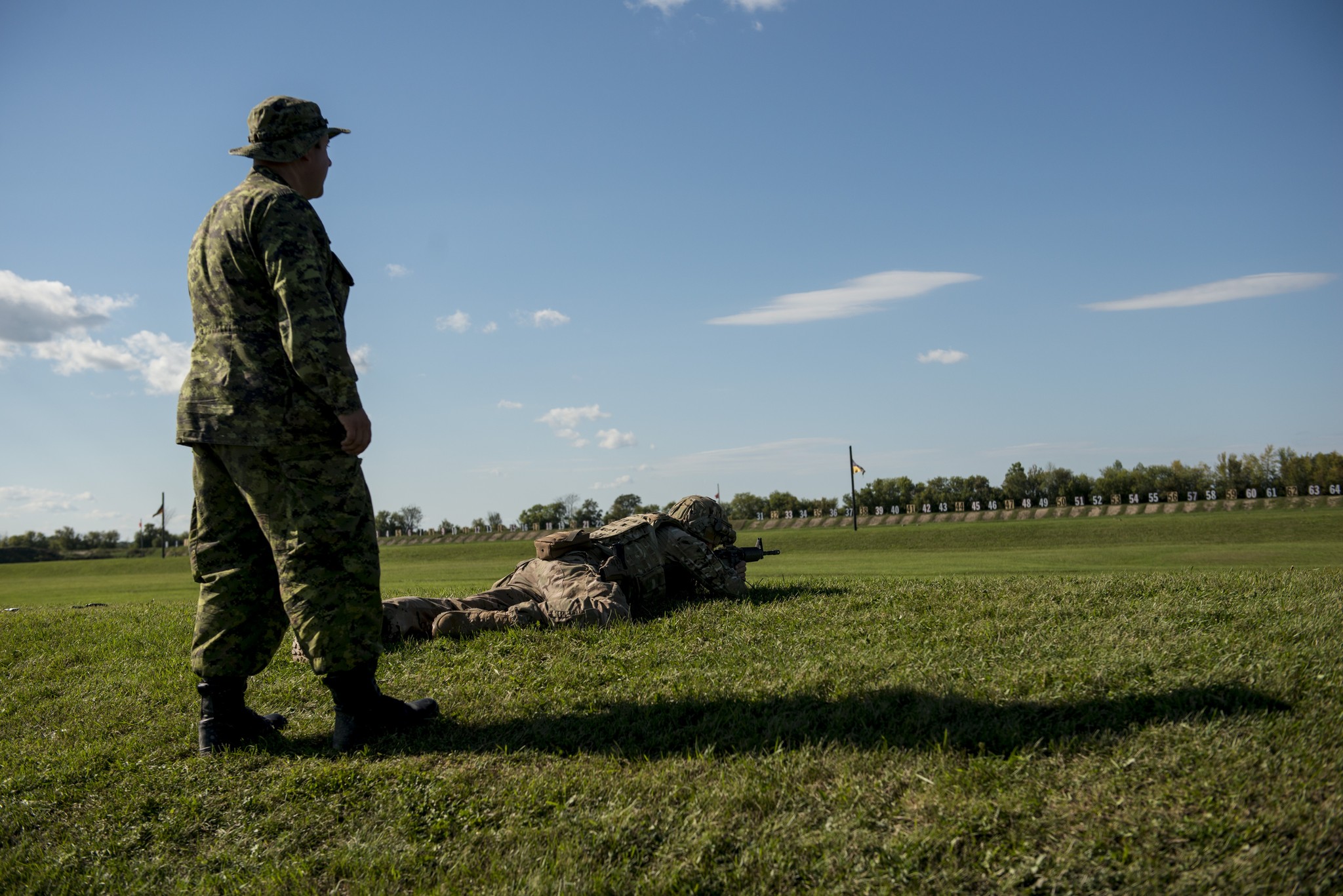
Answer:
227, 96, 351, 164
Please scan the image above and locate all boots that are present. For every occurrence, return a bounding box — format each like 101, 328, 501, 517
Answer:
321, 658, 440, 751
430, 607, 510, 639
198, 680, 288, 757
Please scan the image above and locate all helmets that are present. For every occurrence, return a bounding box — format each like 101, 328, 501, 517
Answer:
667, 496, 729, 546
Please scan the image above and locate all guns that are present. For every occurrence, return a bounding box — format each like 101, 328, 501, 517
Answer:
710, 537, 781, 584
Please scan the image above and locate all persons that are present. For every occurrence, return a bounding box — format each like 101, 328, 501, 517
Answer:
176, 95, 441, 754
293, 495, 748, 662
